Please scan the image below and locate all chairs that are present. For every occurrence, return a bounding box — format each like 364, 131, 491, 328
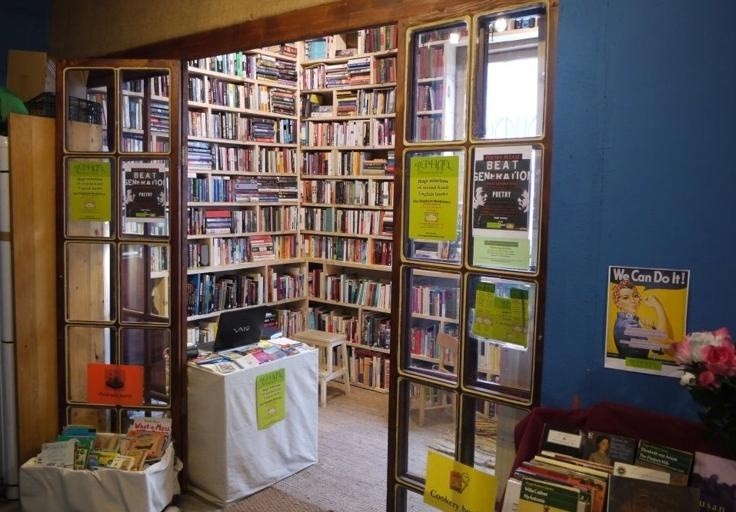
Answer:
408, 332, 461, 426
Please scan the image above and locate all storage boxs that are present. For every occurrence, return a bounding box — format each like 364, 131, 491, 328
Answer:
5, 46, 90, 105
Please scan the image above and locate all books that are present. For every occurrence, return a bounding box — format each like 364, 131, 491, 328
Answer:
499, 420, 736, 510
87, 92, 107, 144
408, 282, 499, 419
414, 22, 468, 142
120, 159, 171, 238
122, 73, 171, 152
488, 16, 539, 31
183, 23, 396, 392
36, 415, 172, 472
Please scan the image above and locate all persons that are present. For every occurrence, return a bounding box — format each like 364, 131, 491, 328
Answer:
509, 189, 529, 228
473, 186, 493, 228
612, 277, 669, 358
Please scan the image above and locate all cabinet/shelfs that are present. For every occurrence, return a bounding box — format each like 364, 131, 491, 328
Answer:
298, 29, 456, 398
90, 38, 306, 346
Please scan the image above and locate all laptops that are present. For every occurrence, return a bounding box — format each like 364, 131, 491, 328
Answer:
196, 305, 268, 353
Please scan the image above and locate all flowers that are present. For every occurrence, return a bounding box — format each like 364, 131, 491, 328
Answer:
660, 324, 736, 447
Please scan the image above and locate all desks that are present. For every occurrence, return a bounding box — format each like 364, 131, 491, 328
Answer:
187, 338, 319, 505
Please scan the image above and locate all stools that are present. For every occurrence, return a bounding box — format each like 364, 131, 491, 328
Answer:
291, 327, 351, 408
